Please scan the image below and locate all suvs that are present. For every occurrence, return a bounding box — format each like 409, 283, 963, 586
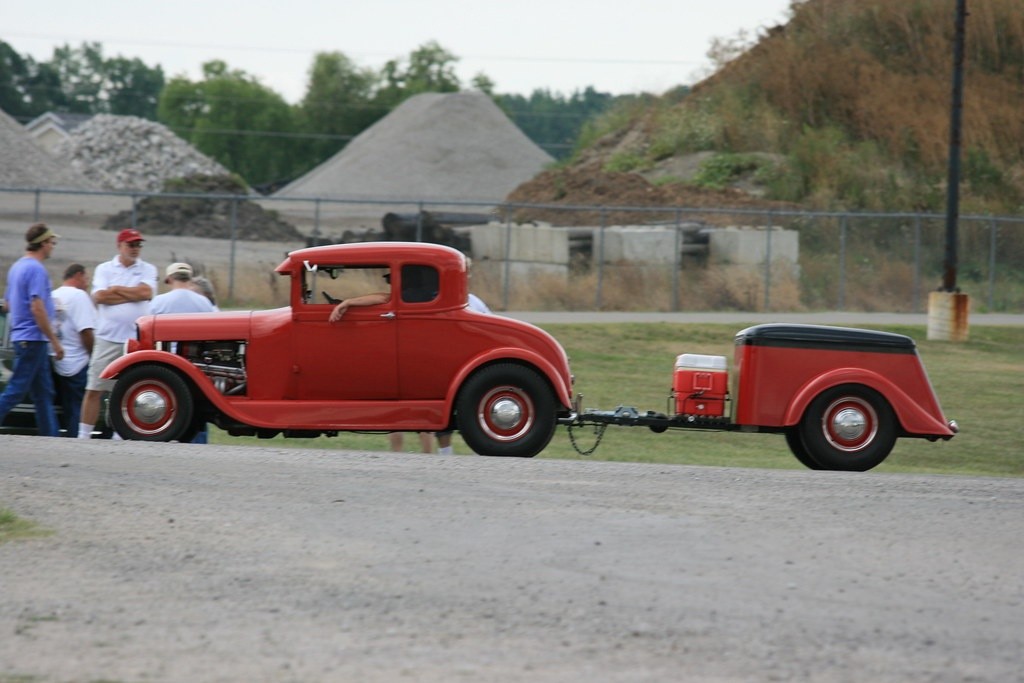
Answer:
99, 242, 574, 458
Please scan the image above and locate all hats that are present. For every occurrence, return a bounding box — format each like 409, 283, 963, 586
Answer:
30, 229, 60, 244
118, 229, 146, 242
164, 262, 192, 283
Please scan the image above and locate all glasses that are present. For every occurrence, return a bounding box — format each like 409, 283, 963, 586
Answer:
128, 242, 144, 249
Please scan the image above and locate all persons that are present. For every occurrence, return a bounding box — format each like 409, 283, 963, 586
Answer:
391, 292, 491, 455
343, 209, 462, 249
329, 267, 392, 322
0, 223, 218, 444
